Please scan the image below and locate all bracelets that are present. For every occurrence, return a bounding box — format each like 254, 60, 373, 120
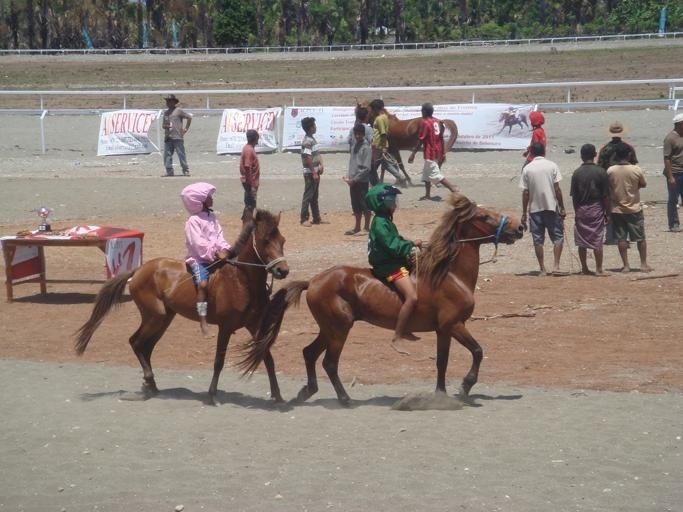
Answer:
183, 129, 188, 132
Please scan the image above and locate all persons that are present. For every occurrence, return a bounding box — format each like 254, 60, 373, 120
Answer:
339, 99, 459, 237
363, 182, 423, 342
180, 182, 231, 335
297, 117, 330, 228
238, 129, 261, 223
661, 112, 682, 231
519, 110, 655, 278
159, 94, 193, 176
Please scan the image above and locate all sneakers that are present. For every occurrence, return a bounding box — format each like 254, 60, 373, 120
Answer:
160, 173, 176, 177
301, 220, 312, 227
670, 226, 682, 232
183, 170, 191, 176
313, 219, 330, 224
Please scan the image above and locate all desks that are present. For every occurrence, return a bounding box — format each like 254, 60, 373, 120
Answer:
0, 224, 144, 302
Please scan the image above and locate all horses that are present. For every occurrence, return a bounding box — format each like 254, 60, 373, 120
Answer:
353, 97, 458, 189
497, 112, 529, 135
68, 208, 287, 407
220, 191, 525, 409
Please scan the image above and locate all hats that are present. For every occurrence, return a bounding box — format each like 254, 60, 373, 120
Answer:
672, 113, 683, 124
163, 94, 179, 104
606, 120, 630, 138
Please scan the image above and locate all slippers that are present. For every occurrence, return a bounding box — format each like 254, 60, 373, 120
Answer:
344, 229, 357, 235
595, 272, 612, 277
354, 230, 370, 236
539, 272, 548, 277
640, 267, 655, 272
552, 269, 570, 276
620, 267, 630, 273
580, 270, 595, 275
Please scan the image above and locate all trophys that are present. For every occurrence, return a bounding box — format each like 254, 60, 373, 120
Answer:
37, 206, 52, 232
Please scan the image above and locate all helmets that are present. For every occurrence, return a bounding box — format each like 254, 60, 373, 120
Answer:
364, 182, 403, 213
367, 98, 385, 108
528, 111, 546, 127
